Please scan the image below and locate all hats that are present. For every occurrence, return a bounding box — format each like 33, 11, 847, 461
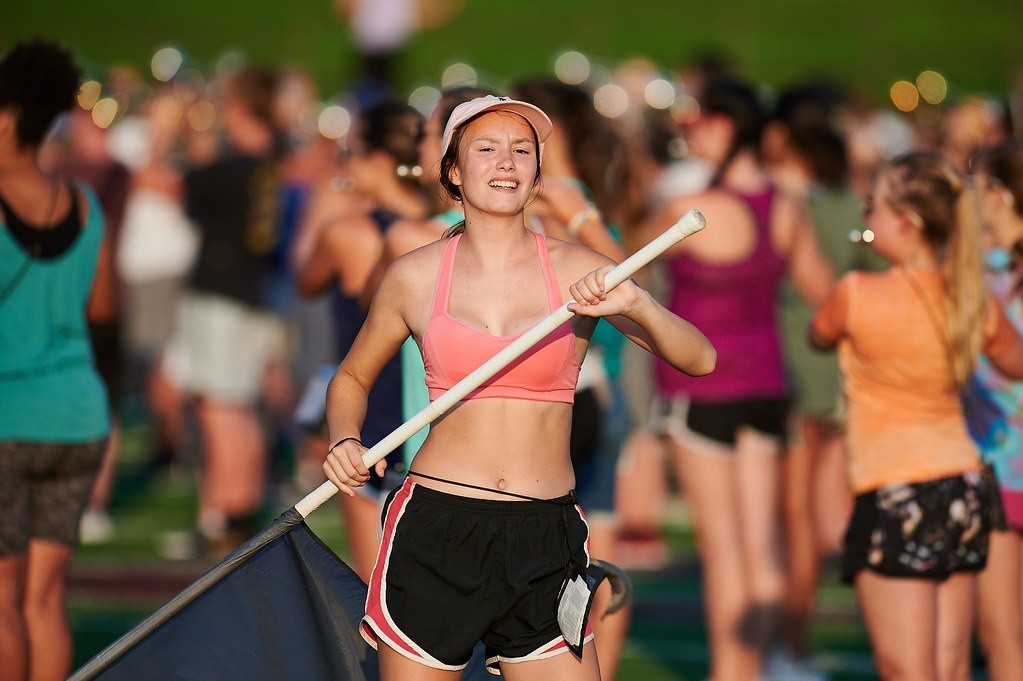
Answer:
442, 94, 552, 167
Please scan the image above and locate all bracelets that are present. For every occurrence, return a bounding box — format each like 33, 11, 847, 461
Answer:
329, 437, 362, 455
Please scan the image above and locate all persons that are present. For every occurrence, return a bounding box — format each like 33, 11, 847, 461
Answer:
1, 35, 112, 681
807, 147, 1023, 681
322, 93, 717, 681
40, 40, 1023, 681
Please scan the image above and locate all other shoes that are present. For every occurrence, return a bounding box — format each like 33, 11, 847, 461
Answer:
734, 575, 785, 645
80, 514, 111, 543
588, 516, 667, 570
158, 530, 194, 562
